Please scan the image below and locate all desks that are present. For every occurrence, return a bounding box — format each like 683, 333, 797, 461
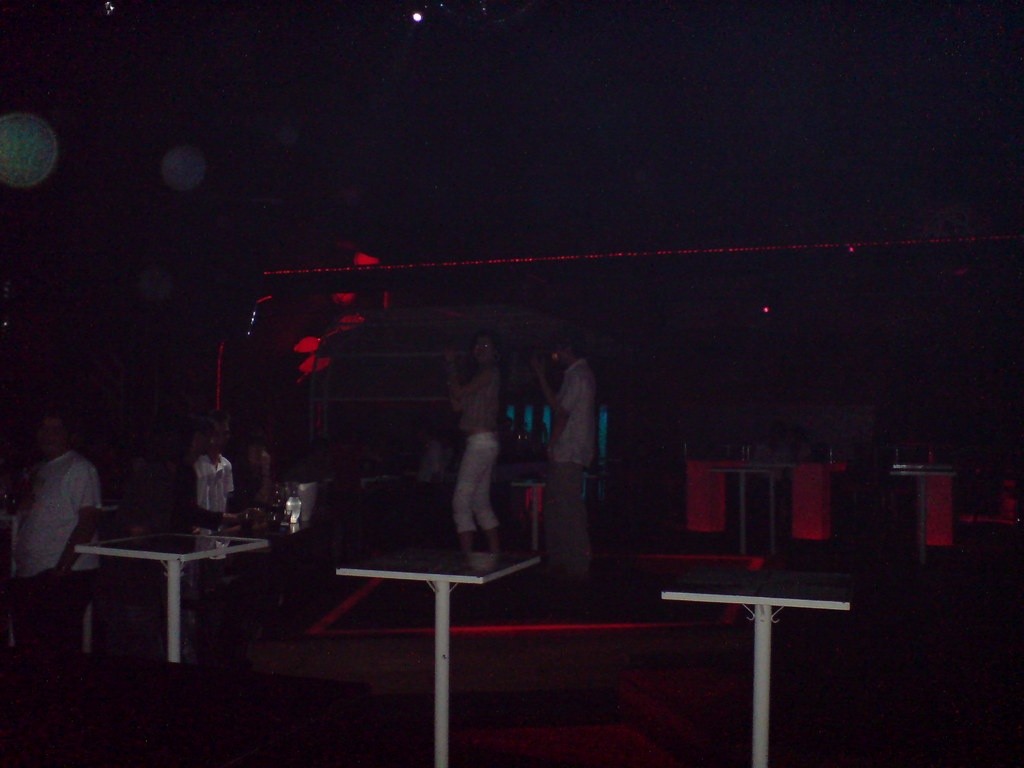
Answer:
889, 464, 958, 565
336, 548, 541, 768
509, 478, 549, 550
74, 532, 270, 665
661, 569, 853, 768
686, 456, 833, 556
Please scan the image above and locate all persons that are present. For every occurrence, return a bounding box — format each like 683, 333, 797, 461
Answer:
0, 328, 597, 676
